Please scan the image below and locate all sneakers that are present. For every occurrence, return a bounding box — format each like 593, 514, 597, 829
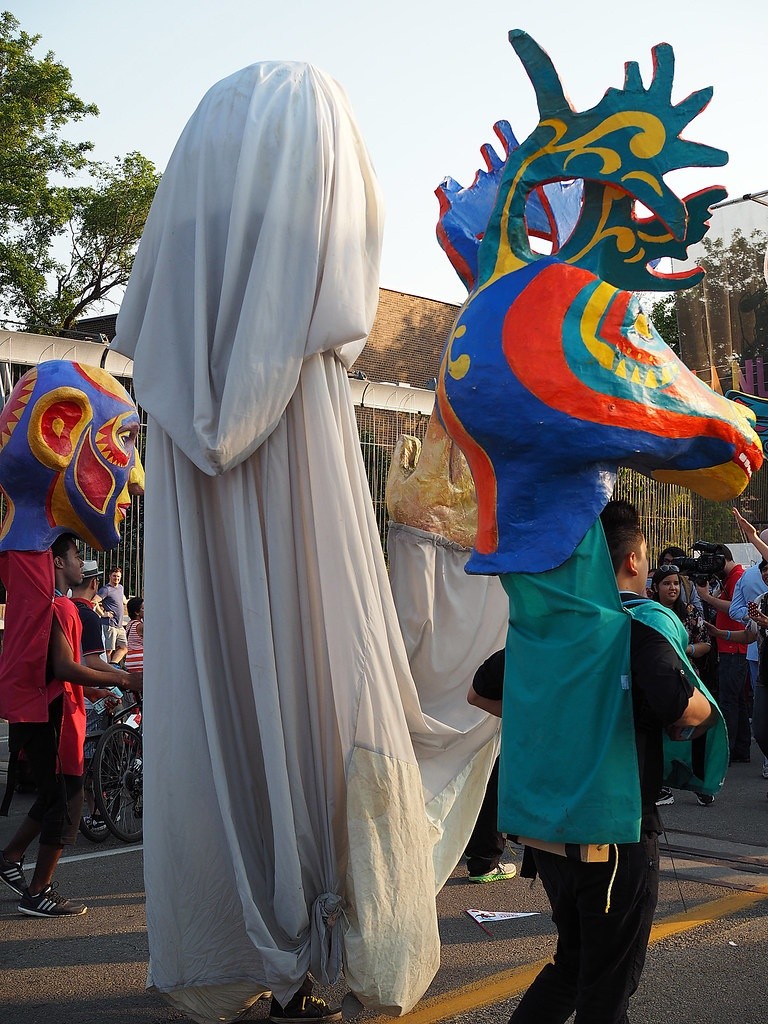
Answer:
0, 849, 28, 897
17, 881, 87, 918
268, 992, 341, 1023
655, 788, 674, 805
469, 864, 516, 883
695, 792, 714, 806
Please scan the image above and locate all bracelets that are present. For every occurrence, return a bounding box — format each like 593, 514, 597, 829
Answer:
690, 644, 695, 656
725, 630, 730, 641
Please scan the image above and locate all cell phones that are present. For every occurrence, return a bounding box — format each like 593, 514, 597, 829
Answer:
747, 601, 759, 618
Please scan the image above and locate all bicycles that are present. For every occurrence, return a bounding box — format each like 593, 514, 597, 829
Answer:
65, 657, 144, 845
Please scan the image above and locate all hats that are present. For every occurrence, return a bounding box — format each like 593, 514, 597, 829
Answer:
82, 559, 104, 577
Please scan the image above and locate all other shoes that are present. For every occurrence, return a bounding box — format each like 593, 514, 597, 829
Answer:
762, 762, 768, 778
77, 817, 106, 834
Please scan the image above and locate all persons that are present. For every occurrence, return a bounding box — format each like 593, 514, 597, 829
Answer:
262, 975, 343, 1023
467, 501, 712, 1024
647, 507, 768, 806
0, 534, 144, 918
464, 756, 517, 884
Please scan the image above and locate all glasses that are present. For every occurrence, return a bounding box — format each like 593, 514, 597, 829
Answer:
659, 564, 680, 573
